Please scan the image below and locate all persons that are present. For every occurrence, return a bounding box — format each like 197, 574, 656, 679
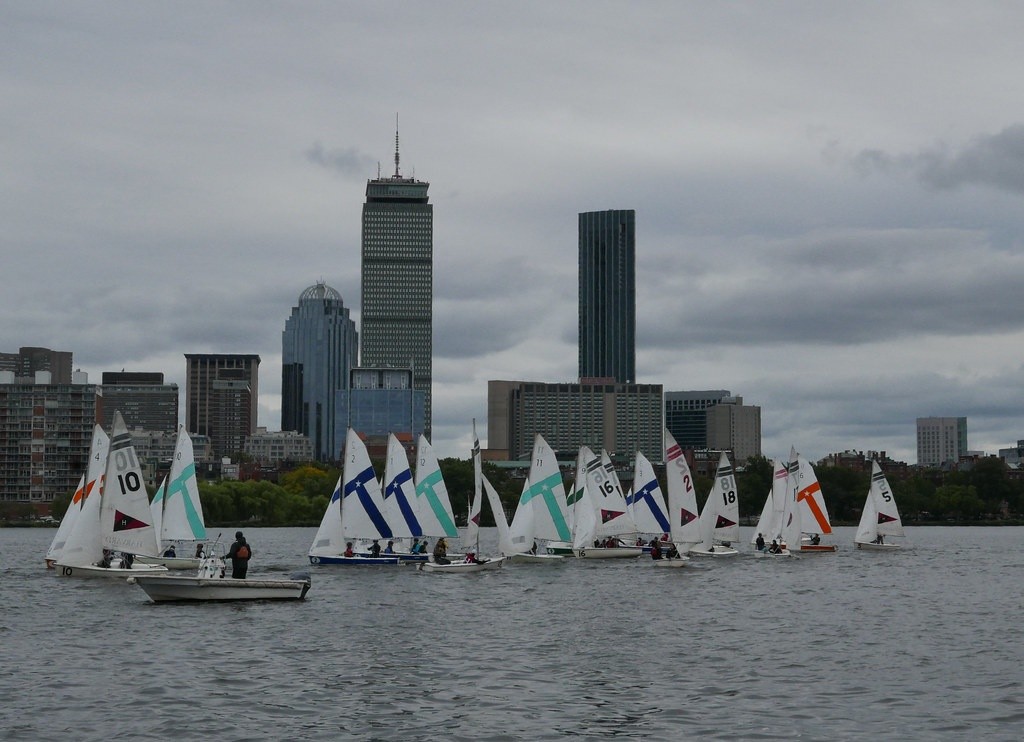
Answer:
419, 541, 429, 570
163, 545, 176, 557
344, 542, 353, 557
411, 538, 421, 554
220, 532, 253, 578
368, 539, 381, 557
594, 532, 886, 561
433, 537, 451, 565
196, 543, 205, 558
384, 541, 395, 553
96, 548, 137, 569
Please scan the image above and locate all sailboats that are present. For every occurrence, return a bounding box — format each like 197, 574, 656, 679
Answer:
53, 410, 170, 580
853, 460, 906, 551
353, 428, 837, 569
44, 422, 112, 569
128, 423, 226, 568
308, 428, 406, 567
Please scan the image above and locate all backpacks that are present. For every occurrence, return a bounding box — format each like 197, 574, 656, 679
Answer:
236, 544, 249, 559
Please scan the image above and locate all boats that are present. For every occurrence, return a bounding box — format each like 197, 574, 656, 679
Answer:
134, 560, 312, 606
